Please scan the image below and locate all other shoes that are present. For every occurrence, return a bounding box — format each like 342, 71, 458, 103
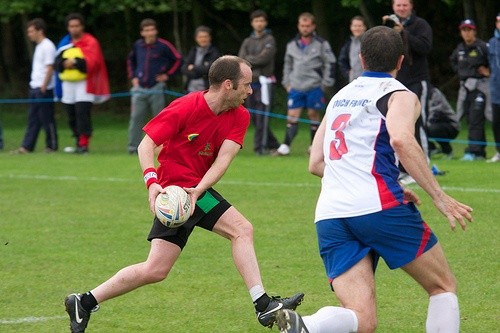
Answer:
18, 147, 31, 153
487, 152, 500, 163
431, 166, 446, 176
129, 150, 138, 154
308, 145, 313, 154
271, 143, 290, 155
429, 149, 439, 155
445, 150, 453, 157
463, 153, 476, 161
75, 146, 88, 153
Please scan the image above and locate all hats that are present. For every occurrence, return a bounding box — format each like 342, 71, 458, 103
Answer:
459, 19, 477, 29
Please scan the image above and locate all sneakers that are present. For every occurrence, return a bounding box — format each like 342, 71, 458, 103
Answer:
276, 308, 310, 333
64, 293, 99, 333
255, 292, 304, 328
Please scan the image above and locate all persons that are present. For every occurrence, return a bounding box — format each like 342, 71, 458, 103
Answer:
486, 15, 500, 163
237, 10, 280, 156
338, 17, 370, 85
427, 87, 461, 159
55, 14, 110, 154
276, 26, 473, 333
382, 1, 445, 177
276, 11, 336, 157
179, 25, 222, 92
8, 18, 59, 154
61, 55, 307, 333
449, 18, 490, 163
124, 19, 183, 157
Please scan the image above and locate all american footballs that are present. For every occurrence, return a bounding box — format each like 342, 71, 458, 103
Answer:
153, 184, 192, 228
